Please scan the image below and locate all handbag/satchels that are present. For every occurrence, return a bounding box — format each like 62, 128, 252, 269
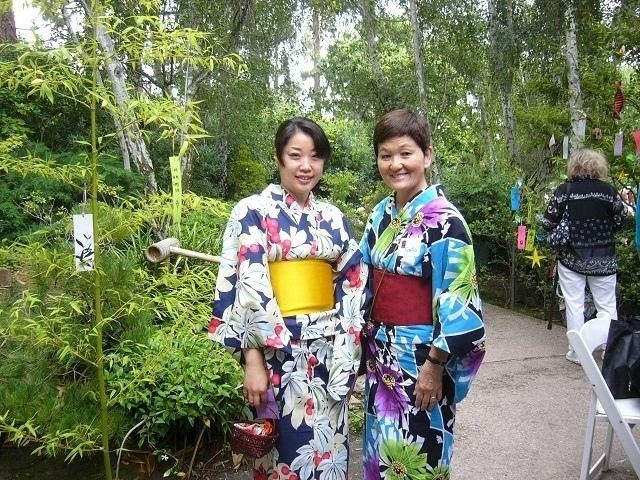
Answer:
600, 316, 640, 400
545, 204, 574, 249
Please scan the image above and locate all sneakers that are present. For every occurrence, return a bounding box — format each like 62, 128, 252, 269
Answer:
565, 349, 581, 366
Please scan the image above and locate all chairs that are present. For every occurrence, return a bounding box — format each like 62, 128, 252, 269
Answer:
564, 311, 639, 479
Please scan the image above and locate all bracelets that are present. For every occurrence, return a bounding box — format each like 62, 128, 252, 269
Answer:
425, 354, 445, 367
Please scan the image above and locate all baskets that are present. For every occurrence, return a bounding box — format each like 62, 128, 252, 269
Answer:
229, 400, 281, 460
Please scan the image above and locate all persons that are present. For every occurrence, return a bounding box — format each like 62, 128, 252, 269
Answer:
207, 118, 362, 480
542, 147, 629, 367
359, 106, 484, 480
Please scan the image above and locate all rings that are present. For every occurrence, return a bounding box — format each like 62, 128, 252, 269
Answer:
430, 396, 436, 399
